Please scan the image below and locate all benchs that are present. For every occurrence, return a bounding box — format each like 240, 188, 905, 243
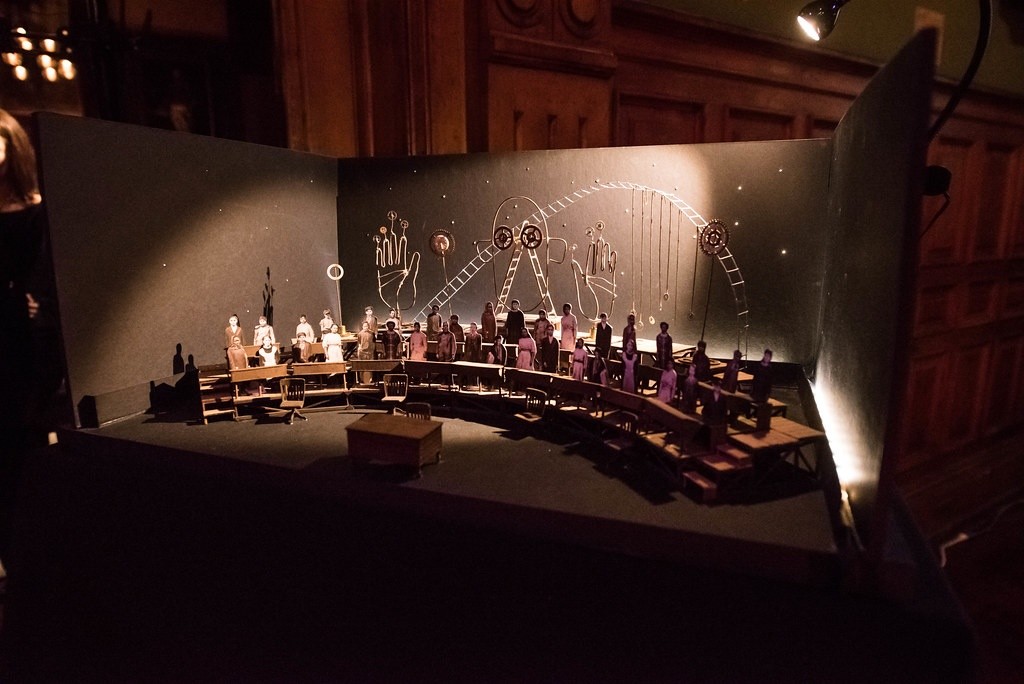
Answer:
232, 327, 826, 506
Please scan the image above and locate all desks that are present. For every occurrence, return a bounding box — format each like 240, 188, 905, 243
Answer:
712, 372, 756, 391
675, 355, 730, 371
495, 309, 566, 337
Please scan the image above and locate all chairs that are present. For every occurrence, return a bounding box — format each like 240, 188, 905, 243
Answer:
403, 401, 433, 420
512, 384, 549, 433
599, 408, 640, 470
278, 375, 308, 426
381, 370, 408, 412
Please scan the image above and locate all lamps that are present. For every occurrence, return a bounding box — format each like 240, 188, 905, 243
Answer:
794, 0, 846, 44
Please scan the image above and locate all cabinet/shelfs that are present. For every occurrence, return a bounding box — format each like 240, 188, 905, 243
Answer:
197, 364, 234, 427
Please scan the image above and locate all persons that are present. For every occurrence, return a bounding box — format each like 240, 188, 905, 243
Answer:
504, 299, 526, 357
253, 309, 344, 381
356, 302, 507, 383
516, 303, 774, 418
227, 335, 249, 369
224, 314, 247, 347
